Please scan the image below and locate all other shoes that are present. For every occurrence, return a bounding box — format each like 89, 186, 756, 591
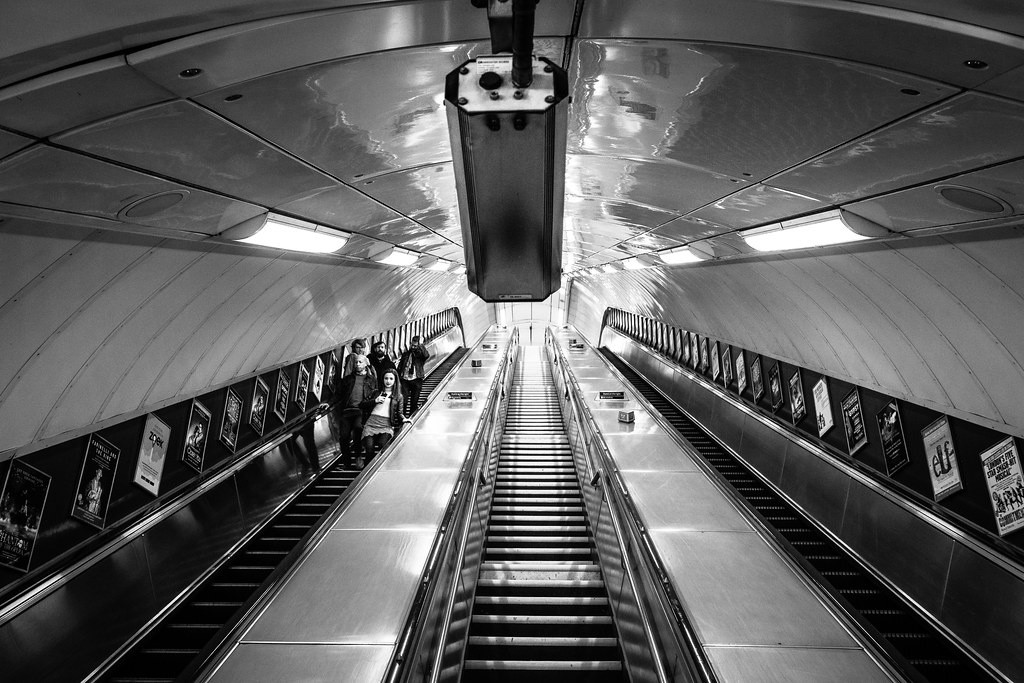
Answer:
341, 465, 353, 471
355, 459, 364, 469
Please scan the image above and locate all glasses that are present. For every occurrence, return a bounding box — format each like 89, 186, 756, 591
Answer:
410, 344, 419, 347
355, 347, 365, 348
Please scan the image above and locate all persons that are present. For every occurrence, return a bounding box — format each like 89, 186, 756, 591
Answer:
334, 354, 380, 470
397, 335, 430, 417
343, 339, 366, 376
367, 342, 397, 391
357, 368, 411, 470
